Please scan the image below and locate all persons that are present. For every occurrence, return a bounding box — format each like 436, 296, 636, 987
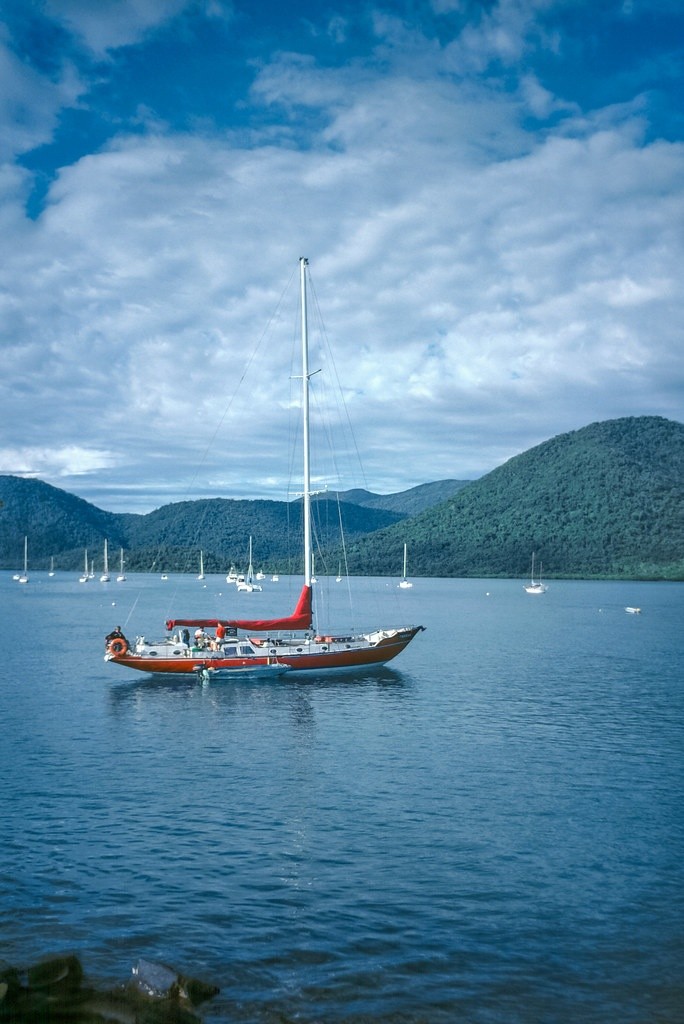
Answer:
105, 626, 126, 639
194, 626, 212, 642
214, 621, 225, 651
182, 628, 190, 648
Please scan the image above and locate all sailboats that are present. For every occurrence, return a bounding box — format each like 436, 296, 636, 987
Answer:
335, 559, 342, 582
116, 547, 128, 581
19, 535, 29, 583
80, 549, 90, 582
197, 550, 206, 579
49, 556, 55, 576
399, 543, 413, 589
89, 560, 95, 579
100, 539, 110, 582
103, 253, 428, 680
522, 551, 546, 594
236, 535, 263, 592
310, 553, 319, 584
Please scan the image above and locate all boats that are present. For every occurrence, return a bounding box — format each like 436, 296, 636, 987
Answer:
225, 561, 239, 584
13, 574, 20, 580
256, 570, 266, 580
160, 574, 169, 580
625, 607, 641, 614
193, 664, 292, 680
270, 574, 279, 581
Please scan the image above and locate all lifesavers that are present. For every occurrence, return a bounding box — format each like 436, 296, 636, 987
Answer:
110, 638, 128, 656
